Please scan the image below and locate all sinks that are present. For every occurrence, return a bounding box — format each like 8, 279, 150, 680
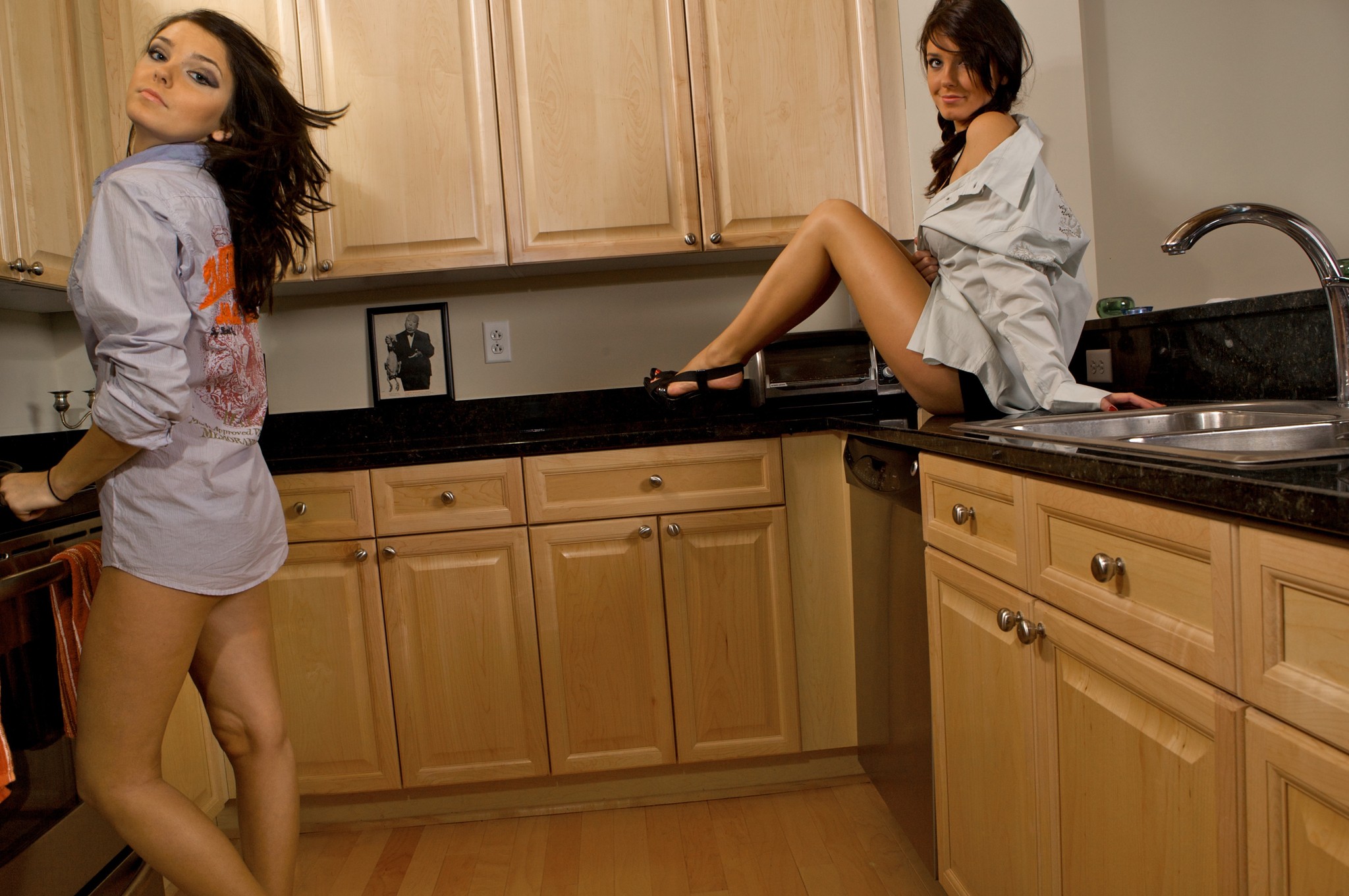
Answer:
1106, 419, 1347, 467
999, 406, 1338, 447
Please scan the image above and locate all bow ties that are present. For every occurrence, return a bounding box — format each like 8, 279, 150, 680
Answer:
405, 331, 414, 337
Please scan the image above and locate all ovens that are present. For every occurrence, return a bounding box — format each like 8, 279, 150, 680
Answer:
0, 561, 166, 896
748, 327, 905, 409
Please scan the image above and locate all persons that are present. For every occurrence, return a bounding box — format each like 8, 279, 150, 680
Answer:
386, 313, 434, 391
0, 10, 352, 896
644, 0, 1165, 419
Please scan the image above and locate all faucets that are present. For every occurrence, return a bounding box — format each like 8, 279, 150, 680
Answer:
1159, 201, 1349, 404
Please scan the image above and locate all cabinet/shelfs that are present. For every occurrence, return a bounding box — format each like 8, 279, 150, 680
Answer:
488, 0, 883, 276
523, 436, 802, 775
269, 457, 548, 796
0, 1, 87, 314
100, 0, 508, 296
917, 453, 1242, 896
162, 675, 230, 826
1241, 520, 1348, 896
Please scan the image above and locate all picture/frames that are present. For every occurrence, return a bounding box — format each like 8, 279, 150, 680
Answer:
366, 301, 454, 407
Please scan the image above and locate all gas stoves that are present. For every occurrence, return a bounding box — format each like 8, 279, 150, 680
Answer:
0, 458, 99, 583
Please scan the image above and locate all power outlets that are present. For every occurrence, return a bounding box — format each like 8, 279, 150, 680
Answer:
1086, 348, 1112, 383
482, 320, 513, 364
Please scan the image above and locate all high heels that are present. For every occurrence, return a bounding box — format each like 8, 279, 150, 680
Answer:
644, 362, 752, 411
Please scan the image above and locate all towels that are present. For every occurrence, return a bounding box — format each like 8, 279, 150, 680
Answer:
45, 539, 104, 739
0, 724, 17, 803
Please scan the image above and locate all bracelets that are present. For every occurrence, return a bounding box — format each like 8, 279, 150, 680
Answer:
47, 469, 67, 502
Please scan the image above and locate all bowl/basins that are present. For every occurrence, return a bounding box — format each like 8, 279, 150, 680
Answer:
1096, 297, 1135, 319
1121, 306, 1153, 316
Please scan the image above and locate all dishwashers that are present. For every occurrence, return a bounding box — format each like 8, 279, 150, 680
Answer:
842, 433, 939, 880
